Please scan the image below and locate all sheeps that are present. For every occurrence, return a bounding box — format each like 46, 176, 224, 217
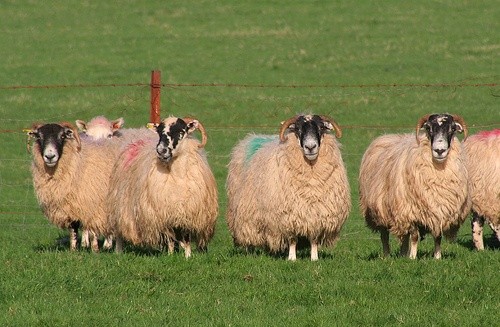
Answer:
360, 112, 500, 260
225, 114, 352, 262
23, 114, 219, 260
